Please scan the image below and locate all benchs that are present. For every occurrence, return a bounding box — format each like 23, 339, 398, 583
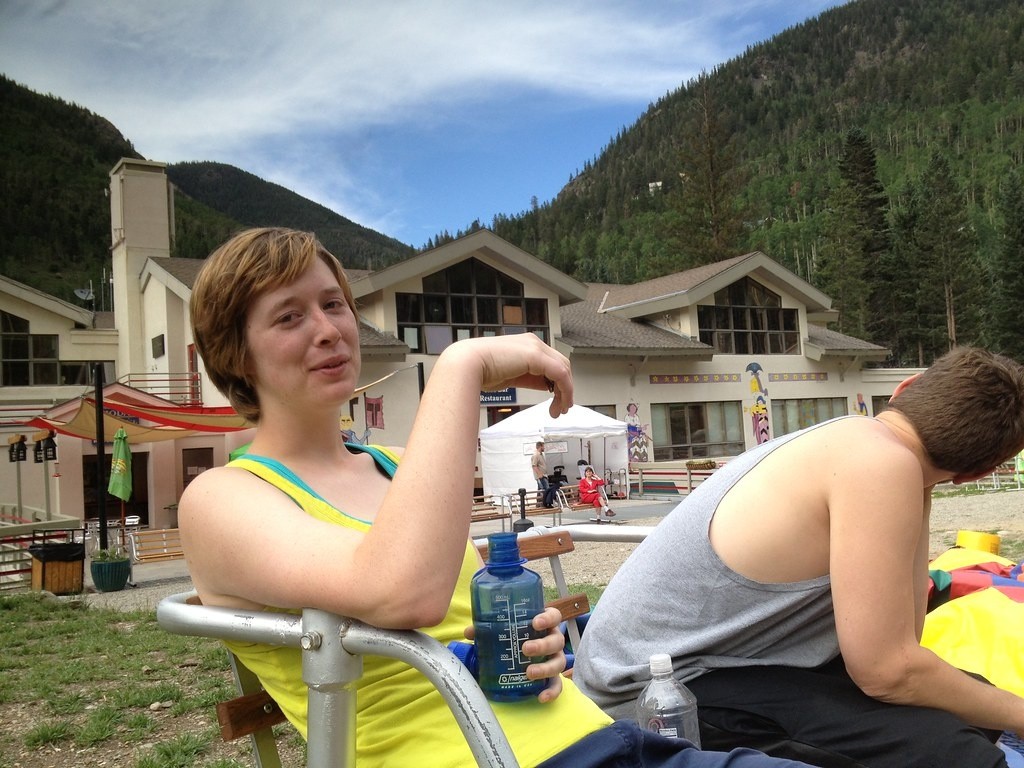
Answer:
127, 527, 184, 586
156, 522, 657, 768
558, 484, 610, 523
503, 489, 563, 527
472, 494, 512, 533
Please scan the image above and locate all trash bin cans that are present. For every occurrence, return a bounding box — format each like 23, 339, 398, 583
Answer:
26, 526, 87, 596
163, 504, 178, 529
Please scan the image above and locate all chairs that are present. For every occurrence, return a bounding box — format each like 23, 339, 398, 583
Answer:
73, 515, 142, 560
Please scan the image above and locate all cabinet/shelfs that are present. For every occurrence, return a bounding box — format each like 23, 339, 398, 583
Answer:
604, 468, 627, 500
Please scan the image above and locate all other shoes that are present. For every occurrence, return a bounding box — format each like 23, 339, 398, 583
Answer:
596, 519, 602, 525
605, 509, 616, 516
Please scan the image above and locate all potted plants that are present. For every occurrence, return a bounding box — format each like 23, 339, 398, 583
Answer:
686, 459, 716, 470
90, 544, 130, 593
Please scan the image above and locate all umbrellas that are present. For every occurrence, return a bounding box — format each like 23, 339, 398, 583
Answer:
108, 427, 133, 553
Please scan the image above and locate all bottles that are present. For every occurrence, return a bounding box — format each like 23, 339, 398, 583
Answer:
635, 653, 700, 751
470, 532, 551, 703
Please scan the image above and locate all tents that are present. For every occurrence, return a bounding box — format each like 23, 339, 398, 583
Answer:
480, 397, 631, 507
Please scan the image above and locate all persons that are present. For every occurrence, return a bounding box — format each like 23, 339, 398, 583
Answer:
178, 227, 813, 768
580, 467, 616, 525
531, 441, 549, 508
568, 347, 1024, 768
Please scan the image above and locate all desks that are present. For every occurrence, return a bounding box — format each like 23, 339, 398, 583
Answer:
83, 518, 124, 554
